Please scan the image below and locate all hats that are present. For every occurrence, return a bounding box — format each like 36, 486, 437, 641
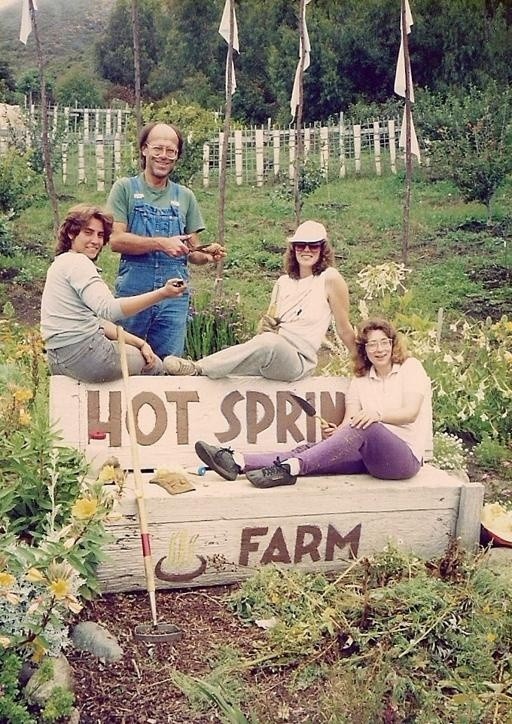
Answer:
287, 220, 328, 242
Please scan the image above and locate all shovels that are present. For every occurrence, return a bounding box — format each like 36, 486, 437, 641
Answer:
290, 395, 329, 429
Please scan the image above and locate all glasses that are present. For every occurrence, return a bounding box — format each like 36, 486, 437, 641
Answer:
364, 338, 392, 353
145, 142, 180, 160
290, 239, 326, 252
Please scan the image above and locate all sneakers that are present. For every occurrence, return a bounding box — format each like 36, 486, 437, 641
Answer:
245, 456, 297, 488
195, 440, 241, 481
164, 354, 198, 375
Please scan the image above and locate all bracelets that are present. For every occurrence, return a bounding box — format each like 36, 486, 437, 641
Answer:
374, 409, 383, 423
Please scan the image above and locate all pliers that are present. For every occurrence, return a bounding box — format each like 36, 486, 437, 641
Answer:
182, 235, 211, 255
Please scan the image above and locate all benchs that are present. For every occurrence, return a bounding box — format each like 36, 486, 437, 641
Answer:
48, 374, 485, 594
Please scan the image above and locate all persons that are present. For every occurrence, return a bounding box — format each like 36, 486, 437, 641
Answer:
194, 319, 433, 489
104, 121, 227, 361
162, 220, 367, 382
40, 206, 188, 384
